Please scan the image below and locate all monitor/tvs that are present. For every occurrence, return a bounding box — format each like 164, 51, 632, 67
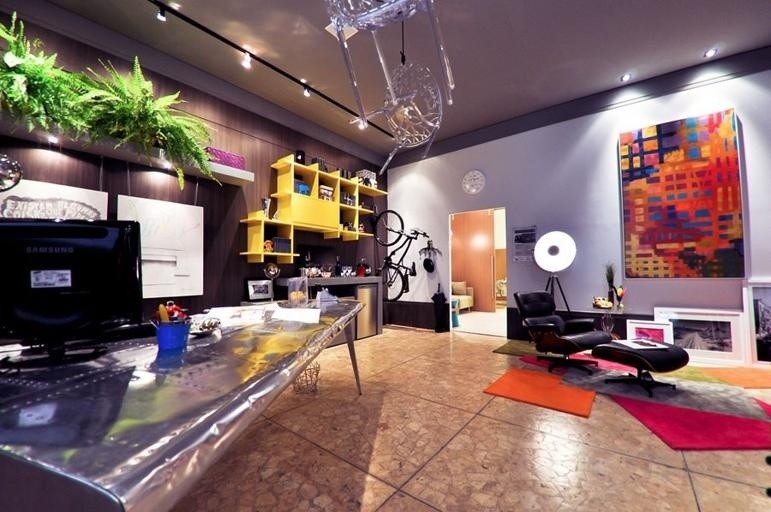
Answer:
0, 217, 144, 368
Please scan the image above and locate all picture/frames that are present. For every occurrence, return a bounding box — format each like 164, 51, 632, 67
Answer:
741, 277, 771, 370
653, 305, 746, 369
625, 319, 674, 347
244, 277, 274, 301
616, 107, 749, 282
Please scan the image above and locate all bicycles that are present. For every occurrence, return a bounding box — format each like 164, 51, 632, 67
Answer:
373, 209, 429, 302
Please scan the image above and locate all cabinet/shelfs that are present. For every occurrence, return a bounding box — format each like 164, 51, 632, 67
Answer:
0, 110, 254, 192
507, 306, 655, 342
278, 275, 383, 340
238, 152, 389, 265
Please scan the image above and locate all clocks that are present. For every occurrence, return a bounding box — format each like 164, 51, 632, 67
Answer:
461, 168, 486, 196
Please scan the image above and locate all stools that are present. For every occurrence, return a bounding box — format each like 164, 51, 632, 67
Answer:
593, 336, 690, 398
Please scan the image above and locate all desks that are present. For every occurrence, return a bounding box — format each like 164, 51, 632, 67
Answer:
0, 298, 368, 511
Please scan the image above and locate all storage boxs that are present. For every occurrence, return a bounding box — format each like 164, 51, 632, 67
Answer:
201, 147, 248, 170
355, 169, 377, 190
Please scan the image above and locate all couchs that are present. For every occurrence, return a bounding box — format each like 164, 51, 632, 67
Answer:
452, 286, 474, 313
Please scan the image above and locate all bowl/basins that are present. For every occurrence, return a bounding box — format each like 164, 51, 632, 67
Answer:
352, 273, 357, 276
322, 273, 331, 278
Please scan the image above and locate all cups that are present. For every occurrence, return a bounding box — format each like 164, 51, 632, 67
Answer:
341, 273, 343, 276
346, 273, 349, 277
348, 266, 353, 275
287, 276, 308, 307
342, 266, 347, 276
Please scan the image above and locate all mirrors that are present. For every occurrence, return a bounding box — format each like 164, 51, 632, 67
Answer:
449, 206, 508, 339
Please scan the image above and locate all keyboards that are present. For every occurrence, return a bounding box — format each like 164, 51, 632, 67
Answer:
105, 322, 159, 341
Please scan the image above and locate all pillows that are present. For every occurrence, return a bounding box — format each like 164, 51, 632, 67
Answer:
452, 282, 466, 295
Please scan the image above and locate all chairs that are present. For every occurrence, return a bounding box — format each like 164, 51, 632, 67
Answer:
513, 290, 613, 375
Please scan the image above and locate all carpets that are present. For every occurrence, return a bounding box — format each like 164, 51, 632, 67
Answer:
482, 338, 770, 452
